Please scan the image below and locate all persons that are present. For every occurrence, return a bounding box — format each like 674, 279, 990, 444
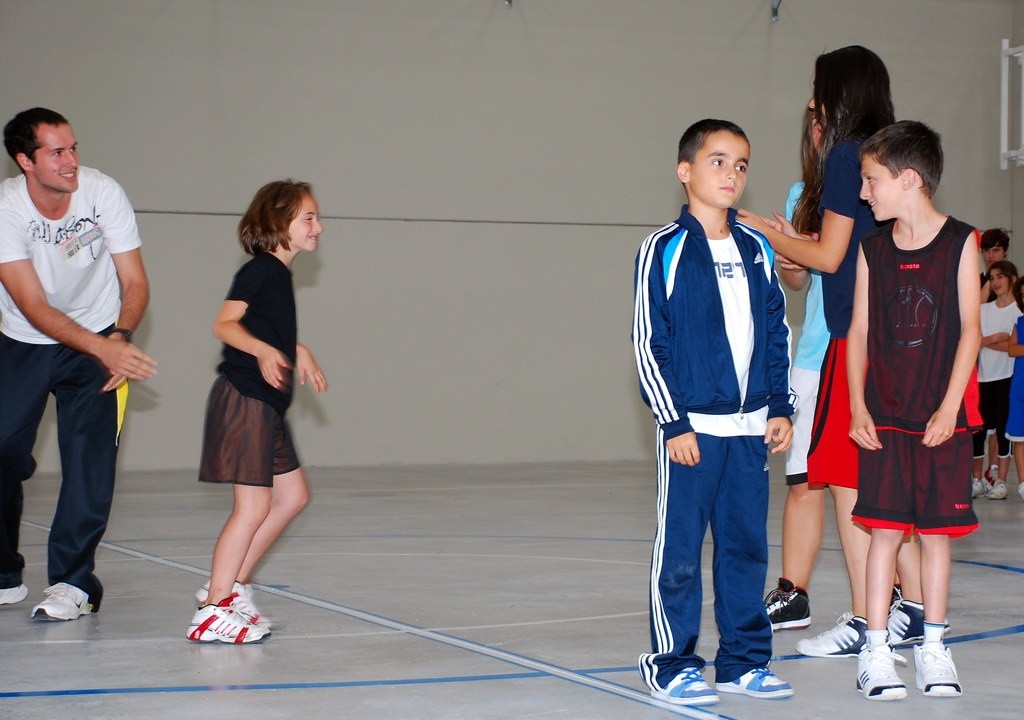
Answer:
765, 99, 903, 629
846, 120, 983, 700
735, 43, 951, 657
188, 179, 329, 644
632, 118, 799, 705
972, 228, 1024, 502
0, 107, 160, 620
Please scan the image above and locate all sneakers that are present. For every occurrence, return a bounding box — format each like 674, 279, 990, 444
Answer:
1017, 481, 1024, 501
889, 584, 905, 605
0, 581, 28, 604
763, 577, 812, 631
186, 592, 272, 643
855, 641, 906, 700
30, 580, 94, 621
651, 665, 719, 705
794, 610, 892, 657
716, 664, 795, 698
986, 482, 1008, 500
911, 641, 963, 697
887, 586, 952, 646
194, 577, 272, 630
984, 465, 998, 487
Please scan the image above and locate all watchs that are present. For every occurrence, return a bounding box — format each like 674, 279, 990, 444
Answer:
111, 328, 132, 342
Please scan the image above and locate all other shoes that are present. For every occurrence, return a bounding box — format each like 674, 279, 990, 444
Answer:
971, 478, 985, 497
